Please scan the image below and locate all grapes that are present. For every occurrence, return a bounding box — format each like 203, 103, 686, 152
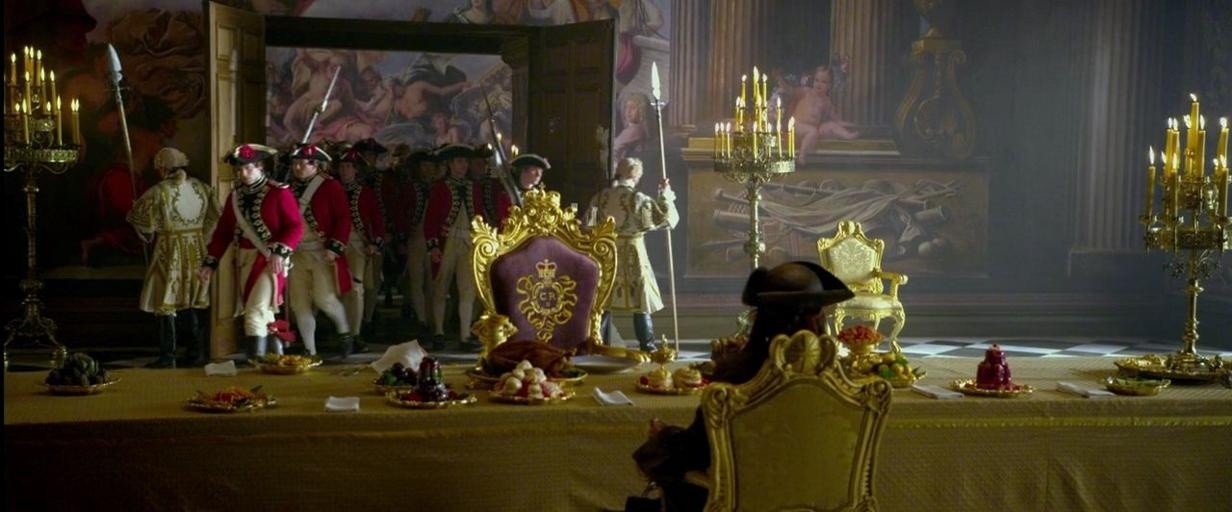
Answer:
417, 383, 446, 401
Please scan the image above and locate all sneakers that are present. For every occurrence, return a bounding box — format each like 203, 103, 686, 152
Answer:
410, 320, 487, 353
336, 294, 395, 358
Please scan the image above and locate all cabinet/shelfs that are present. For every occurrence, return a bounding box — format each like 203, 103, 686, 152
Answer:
679, 135, 991, 296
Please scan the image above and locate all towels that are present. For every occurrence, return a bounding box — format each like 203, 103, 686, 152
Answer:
1057, 379, 1117, 399
366, 337, 431, 374
204, 360, 238, 376
909, 382, 967, 403
590, 385, 635, 406
322, 393, 361, 413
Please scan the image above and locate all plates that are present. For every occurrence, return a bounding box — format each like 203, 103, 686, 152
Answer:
369, 364, 591, 411
181, 390, 278, 412
31, 369, 121, 395
852, 366, 928, 387
632, 371, 709, 395
570, 355, 640, 375
245, 352, 324, 374
948, 372, 1035, 400
1095, 354, 1226, 396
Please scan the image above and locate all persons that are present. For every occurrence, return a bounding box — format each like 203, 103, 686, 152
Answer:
583, 157, 680, 352
443, 0, 496, 25
125, 138, 552, 367
384, 77, 472, 120
777, 66, 862, 166
613, 91, 655, 172
649, 259, 856, 512
432, 113, 466, 146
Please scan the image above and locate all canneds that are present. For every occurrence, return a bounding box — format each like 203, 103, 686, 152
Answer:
420, 360, 440, 387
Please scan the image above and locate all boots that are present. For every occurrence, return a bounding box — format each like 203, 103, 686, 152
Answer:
179, 309, 207, 369
143, 312, 178, 369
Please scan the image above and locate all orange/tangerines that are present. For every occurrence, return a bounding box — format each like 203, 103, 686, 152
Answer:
383, 374, 397, 386
878, 352, 915, 379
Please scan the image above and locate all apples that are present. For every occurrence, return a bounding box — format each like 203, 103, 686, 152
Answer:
495, 360, 564, 399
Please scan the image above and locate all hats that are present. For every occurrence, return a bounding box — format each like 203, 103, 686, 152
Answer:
614, 156, 646, 181
223, 143, 280, 166
335, 137, 497, 162
741, 257, 857, 311
280, 143, 333, 162
153, 147, 192, 173
509, 153, 552, 170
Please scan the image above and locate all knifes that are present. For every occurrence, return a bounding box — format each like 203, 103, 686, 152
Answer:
235, 384, 263, 409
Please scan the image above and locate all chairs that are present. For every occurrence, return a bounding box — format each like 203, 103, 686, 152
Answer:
816, 219, 909, 355
648, 327, 894, 512
472, 179, 651, 364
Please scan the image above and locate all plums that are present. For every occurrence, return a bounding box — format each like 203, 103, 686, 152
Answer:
392, 362, 416, 386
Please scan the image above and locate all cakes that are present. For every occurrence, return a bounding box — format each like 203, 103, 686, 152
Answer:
646, 332, 702, 388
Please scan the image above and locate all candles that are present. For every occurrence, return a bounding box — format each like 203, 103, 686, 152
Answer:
1140, 92, 1230, 219
712, 64, 798, 162
1, 46, 82, 148
